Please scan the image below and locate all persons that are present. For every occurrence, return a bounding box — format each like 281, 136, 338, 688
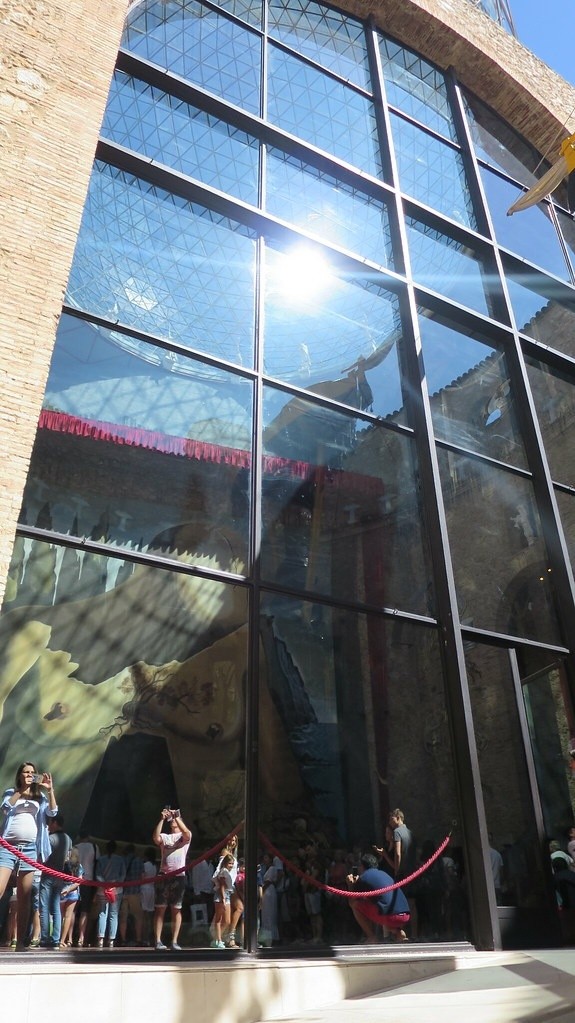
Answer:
548, 735, 575, 947
259, 832, 548, 947
209, 833, 263, 949
373, 809, 421, 944
152, 810, 193, 951
346, 853, 411, 943
0, 812, 233, 947
0, 761, 59, 952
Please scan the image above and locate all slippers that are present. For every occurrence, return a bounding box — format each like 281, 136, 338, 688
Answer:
359, 938, 378, 944
396, 938, 409, 943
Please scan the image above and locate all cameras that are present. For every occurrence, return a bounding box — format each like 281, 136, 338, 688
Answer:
167, 810, 174, 818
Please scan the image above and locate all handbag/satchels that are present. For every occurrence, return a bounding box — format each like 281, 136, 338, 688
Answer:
104, 887, 115, 904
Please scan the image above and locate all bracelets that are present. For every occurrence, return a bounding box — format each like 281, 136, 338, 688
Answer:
47, 789, 55, 793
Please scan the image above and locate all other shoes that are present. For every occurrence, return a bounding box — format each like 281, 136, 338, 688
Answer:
155, 941, 167, 950
170, 941, 182, 951
225, 933, 240, 948
4, 937, 89, 951
210, 939, 226, 949
135, 940, 143, 947
257, 942, 263, 948
120, 939, 127, 947
97, 938, 103, 947
290, 936, 325, 946
108, 940, 114, 948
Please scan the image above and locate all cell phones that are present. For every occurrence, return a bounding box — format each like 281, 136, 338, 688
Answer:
32, 775, 43, 784
352, 867, 357, 880
